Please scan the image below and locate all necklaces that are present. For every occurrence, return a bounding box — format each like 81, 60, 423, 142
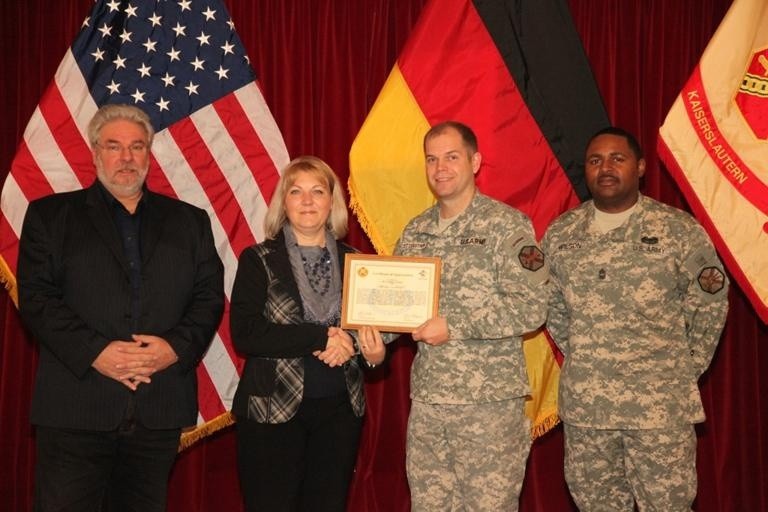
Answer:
296, 243, 332, 295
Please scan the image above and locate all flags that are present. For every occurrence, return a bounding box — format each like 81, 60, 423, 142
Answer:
656, 0, 768, 334
343, 1, 614, 440
0, 0, 295, 449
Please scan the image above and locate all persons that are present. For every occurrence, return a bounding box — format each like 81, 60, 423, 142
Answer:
357, 117, 550, 512
17, 103, 225, 510
228, 156, 392, 510
538, 128, 729, 512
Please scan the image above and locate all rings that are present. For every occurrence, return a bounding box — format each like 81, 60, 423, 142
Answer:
361, 345, 368, 351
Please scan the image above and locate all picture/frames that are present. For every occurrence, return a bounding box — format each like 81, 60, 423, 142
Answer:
339, 253, 442, 332
